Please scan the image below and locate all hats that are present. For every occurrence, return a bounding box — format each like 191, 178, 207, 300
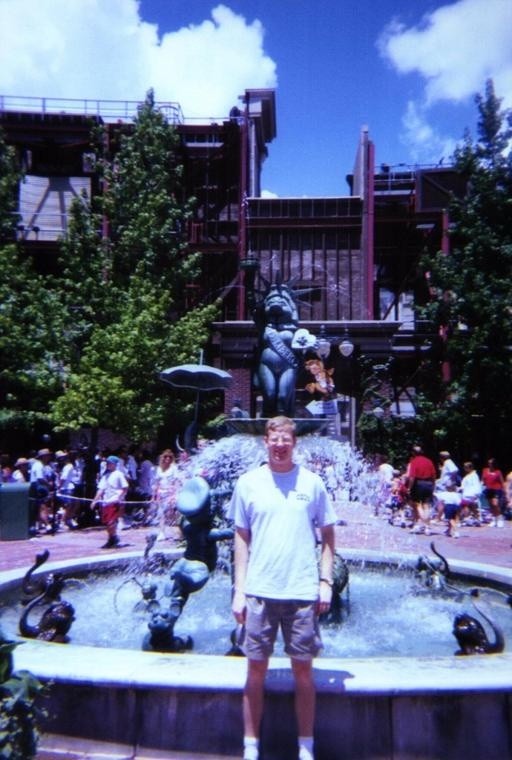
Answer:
14, 443, 88, 468
102, 455, 119, 464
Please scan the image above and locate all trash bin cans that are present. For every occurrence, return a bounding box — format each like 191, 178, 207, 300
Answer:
0, 482, 32, 541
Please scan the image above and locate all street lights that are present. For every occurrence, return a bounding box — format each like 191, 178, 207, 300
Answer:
373, 404, 387, 463
297, 320, 359, 421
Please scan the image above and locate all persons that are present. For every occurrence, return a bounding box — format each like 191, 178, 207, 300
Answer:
308, 445, 512, 538
0, 437, 245, 549
232, 417, 338, 760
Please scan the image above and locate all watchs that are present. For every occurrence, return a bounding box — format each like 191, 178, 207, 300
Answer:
319, 576, 334, 586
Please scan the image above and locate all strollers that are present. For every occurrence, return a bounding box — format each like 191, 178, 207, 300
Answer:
383, 484, 416, 530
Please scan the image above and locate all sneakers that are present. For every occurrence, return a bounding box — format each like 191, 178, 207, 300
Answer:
117, 509, 155, 530
32, 511, 78, 534
102, 535, 118, 549
410, 514, 505, 539
